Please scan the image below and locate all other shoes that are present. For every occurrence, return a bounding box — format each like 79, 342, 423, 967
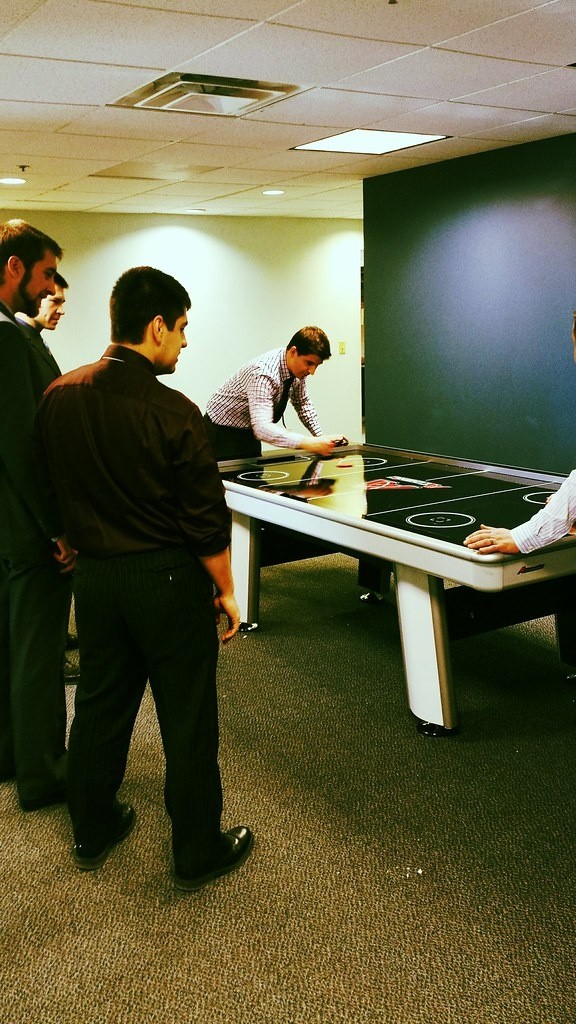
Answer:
63, 660, 83, 684
65, 632, 79, 654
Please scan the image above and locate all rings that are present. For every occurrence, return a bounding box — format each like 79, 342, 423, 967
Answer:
489, 538, 493, 545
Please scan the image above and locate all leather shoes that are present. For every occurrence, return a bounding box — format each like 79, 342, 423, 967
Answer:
173, 823, 255, 898
73, 802, 137, 872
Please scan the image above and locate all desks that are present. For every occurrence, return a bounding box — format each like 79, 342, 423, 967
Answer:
200, 444, 576, 739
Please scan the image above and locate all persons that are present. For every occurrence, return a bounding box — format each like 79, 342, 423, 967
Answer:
31, 264, 258, 894
463, 307, 576, 556
203, 324, 349, 461
0, 219, 85, 813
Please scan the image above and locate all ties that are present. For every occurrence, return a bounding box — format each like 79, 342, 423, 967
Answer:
272, 375, 295, 423
